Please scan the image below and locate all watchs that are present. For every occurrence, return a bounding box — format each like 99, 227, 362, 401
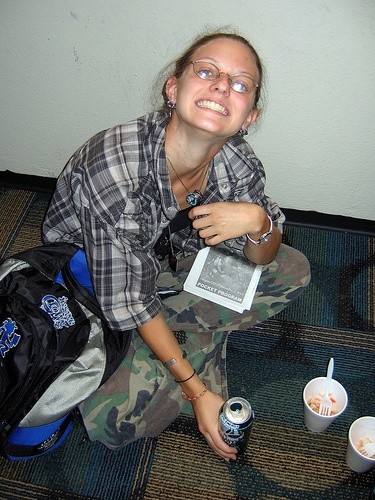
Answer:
246, 215, 273, 246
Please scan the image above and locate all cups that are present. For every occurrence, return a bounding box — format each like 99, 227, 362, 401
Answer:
346, 416, 375, 473
303, 377, 348, 433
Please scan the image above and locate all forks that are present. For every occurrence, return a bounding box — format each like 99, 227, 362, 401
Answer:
318, 357, 334, 416
360, 441, 375, 458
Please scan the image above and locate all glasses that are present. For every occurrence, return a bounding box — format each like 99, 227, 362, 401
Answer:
187, 60, 260, 96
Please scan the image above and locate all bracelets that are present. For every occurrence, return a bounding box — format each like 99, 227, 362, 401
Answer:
175, 369, 196, 383
182, 382, 207, 400
163, 350, 187, 368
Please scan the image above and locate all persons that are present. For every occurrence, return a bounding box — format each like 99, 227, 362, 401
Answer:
43, 24, 311, 463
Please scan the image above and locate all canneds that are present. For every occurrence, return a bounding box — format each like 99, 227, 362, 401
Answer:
218, 397, 254, 457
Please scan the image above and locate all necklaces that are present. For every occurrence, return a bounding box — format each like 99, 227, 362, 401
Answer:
166, 155, 211, 206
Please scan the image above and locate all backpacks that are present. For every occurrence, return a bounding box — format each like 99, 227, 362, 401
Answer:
0, 243, 133, 460
